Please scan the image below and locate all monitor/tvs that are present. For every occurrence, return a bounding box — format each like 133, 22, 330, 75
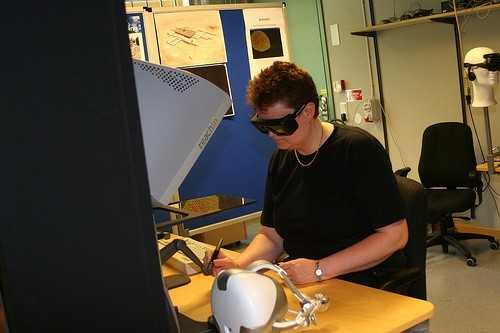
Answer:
132, 57, 232, 206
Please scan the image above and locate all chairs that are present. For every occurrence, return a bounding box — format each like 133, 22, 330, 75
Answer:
367, 175, 429, 301
395, 122, 500, 266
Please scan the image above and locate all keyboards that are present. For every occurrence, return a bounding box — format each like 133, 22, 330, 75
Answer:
158, 236, 216, 275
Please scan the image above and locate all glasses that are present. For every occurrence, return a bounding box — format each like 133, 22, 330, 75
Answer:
250, 103, 307, 136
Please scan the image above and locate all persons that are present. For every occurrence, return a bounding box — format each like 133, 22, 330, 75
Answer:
464, 47, 498, 107
203, 61, 408, 286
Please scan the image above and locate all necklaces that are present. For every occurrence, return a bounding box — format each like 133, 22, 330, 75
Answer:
294, 126, 323, 166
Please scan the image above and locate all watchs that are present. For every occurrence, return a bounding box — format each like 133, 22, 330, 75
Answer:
314, 259, 323, 282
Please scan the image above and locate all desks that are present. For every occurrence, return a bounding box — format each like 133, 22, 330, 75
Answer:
157, 230, 434, 333
